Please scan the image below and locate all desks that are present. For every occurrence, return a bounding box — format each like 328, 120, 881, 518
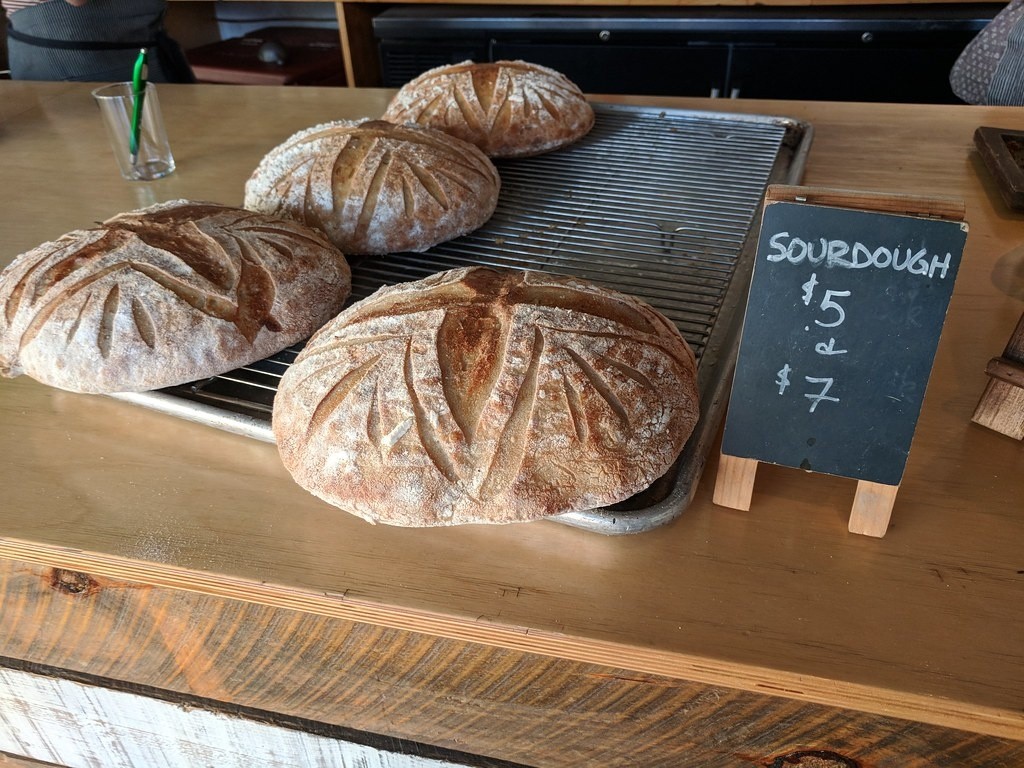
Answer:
1, 79, 1024, 768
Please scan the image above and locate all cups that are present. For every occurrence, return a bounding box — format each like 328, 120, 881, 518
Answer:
91, 82, 177, 181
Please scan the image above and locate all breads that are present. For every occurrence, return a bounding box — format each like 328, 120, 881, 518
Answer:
0, 197, 349, 384
396, 59, 590, 150
262, 119, 496, 246
272, 264, 697, 525
